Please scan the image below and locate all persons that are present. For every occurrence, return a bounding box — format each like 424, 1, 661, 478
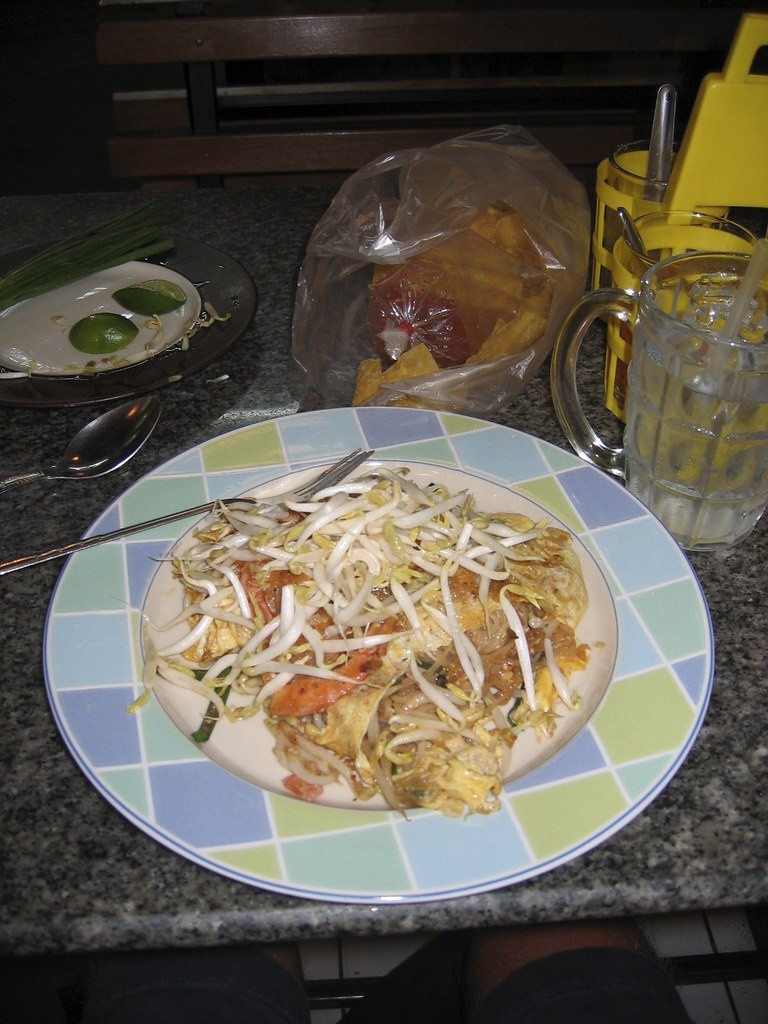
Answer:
82, 916, 693, 1024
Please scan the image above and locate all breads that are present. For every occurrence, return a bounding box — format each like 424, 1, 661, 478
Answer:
371, 194, 554, 375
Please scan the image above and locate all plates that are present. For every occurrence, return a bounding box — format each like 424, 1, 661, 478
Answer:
44, 405, 715, 905
0, 237, 257, 408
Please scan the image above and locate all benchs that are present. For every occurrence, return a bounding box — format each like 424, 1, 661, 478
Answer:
95, 0, 740, 194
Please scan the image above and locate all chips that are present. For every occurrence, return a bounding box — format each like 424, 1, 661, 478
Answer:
347, 338, 466, 409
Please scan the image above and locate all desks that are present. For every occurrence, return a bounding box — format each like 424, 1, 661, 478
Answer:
0, 188, 768, 953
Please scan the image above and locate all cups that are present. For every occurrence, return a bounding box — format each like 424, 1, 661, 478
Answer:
611, 211, 762, 412
600, 140, 680, 288
550, 251, 768, 552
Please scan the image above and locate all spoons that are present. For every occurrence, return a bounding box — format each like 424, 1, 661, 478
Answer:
0, 396, 161, 494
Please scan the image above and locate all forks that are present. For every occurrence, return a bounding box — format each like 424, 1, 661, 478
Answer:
1, 448, 376, 579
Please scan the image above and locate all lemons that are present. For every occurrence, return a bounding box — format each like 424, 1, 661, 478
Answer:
69, 279, 188, 353
